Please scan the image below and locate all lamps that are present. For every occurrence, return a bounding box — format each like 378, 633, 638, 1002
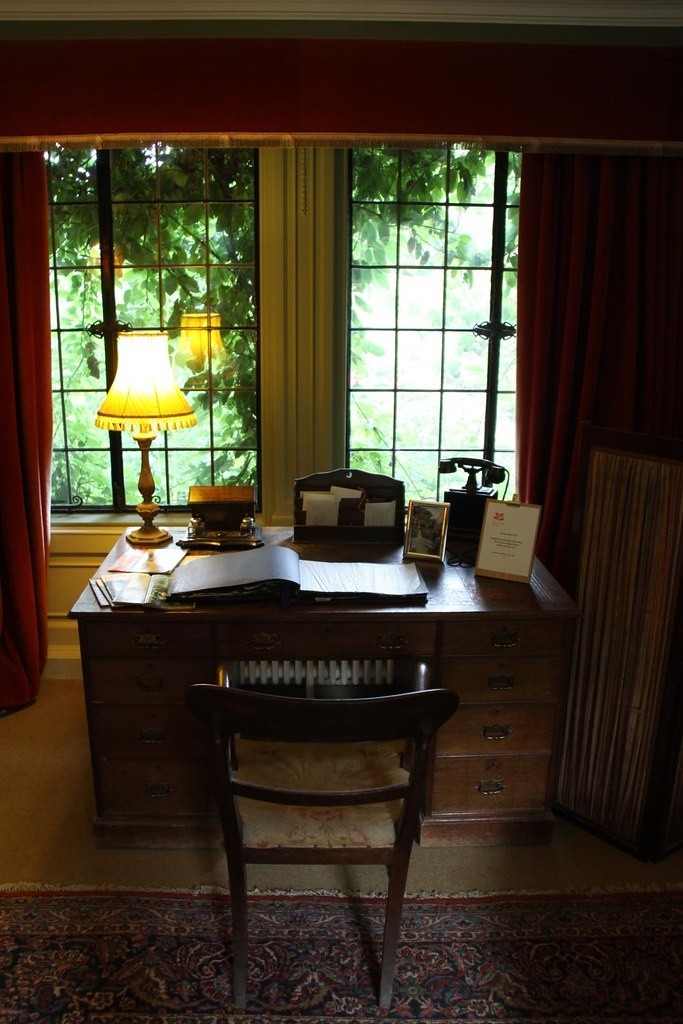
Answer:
173, 313, 234, 386
94, 329, 198, 546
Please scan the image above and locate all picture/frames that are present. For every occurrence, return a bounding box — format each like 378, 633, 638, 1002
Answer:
403, 500, 451, 562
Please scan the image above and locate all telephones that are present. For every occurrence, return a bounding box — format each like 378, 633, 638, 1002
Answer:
437, 456, 510, 567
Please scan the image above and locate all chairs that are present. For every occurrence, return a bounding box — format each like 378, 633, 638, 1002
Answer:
186, 660, 459, 1009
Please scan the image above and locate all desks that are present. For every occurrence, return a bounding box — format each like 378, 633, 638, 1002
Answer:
66, 525, 582, 850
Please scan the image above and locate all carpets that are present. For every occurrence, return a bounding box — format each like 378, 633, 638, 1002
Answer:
0, 878, 682, 1024
